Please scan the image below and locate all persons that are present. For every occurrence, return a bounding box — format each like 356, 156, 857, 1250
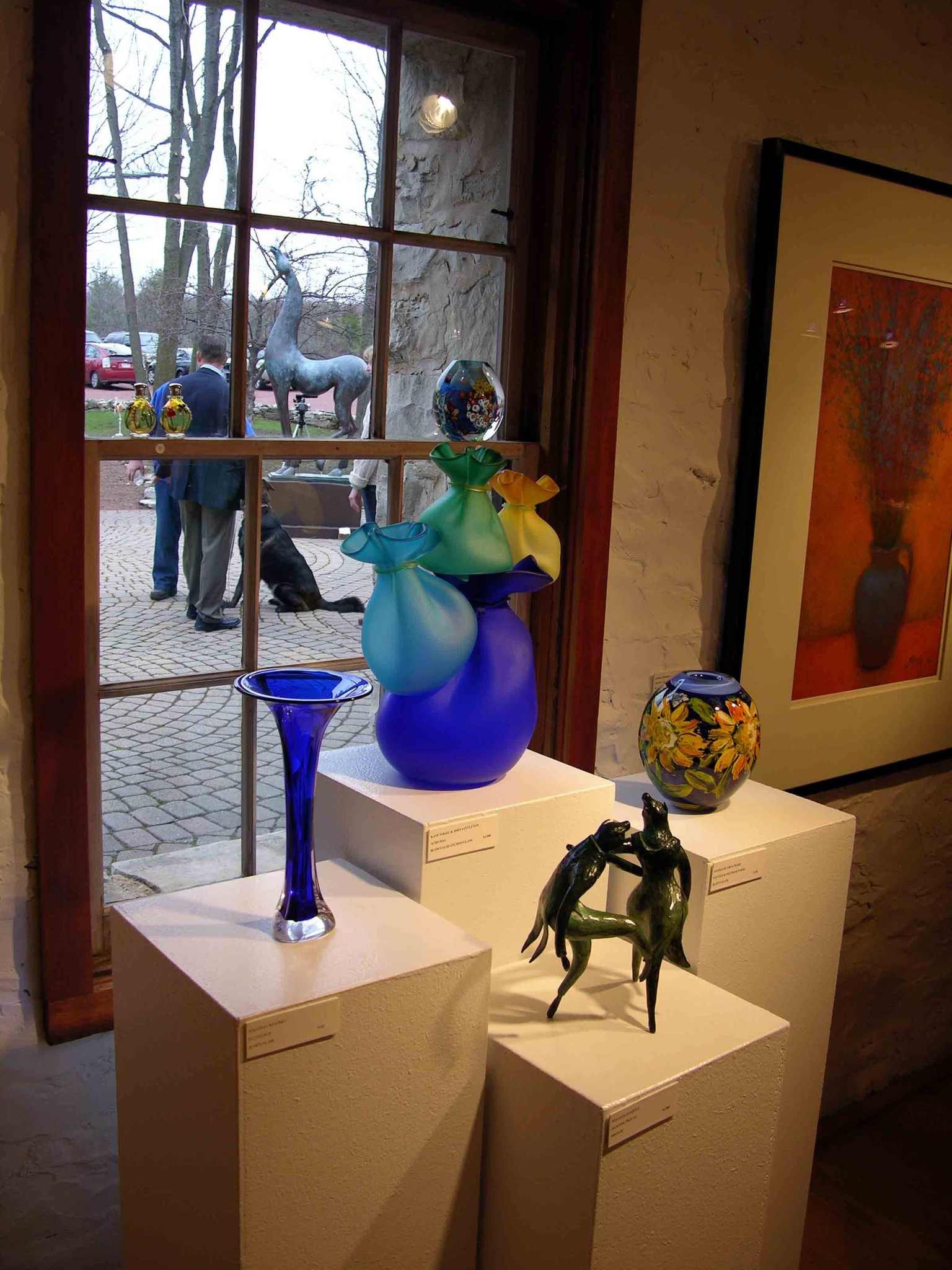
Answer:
348, 344, 378, 626
127, 332, 260, 633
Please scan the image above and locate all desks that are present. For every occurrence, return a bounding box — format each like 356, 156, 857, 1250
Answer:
111, 740, 855, 1270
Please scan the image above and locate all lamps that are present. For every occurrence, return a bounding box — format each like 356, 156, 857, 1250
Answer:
417, 94, 458, 134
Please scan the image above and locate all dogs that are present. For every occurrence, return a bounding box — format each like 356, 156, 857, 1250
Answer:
222, 479, 366, 613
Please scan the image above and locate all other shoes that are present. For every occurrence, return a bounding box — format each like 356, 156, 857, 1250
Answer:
151, 586, 177, 600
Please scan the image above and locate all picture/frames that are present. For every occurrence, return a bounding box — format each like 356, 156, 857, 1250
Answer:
714, 136, 952, 800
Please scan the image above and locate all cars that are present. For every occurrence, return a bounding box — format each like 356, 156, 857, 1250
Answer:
145, 347, 231, 383
227, 342, 271, 390
84, 342, 136, 389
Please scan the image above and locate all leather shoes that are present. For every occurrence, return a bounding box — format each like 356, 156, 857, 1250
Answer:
186, 600, 224, 619
195, 617, 240, 632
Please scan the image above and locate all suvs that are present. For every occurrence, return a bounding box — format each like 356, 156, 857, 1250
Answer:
85, 330, 108, 344
105, 330, 158, 354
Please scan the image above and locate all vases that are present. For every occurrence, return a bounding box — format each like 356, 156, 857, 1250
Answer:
343, 360, 560, 791
233, 667, 372, 942
638, 670, 761, 815
